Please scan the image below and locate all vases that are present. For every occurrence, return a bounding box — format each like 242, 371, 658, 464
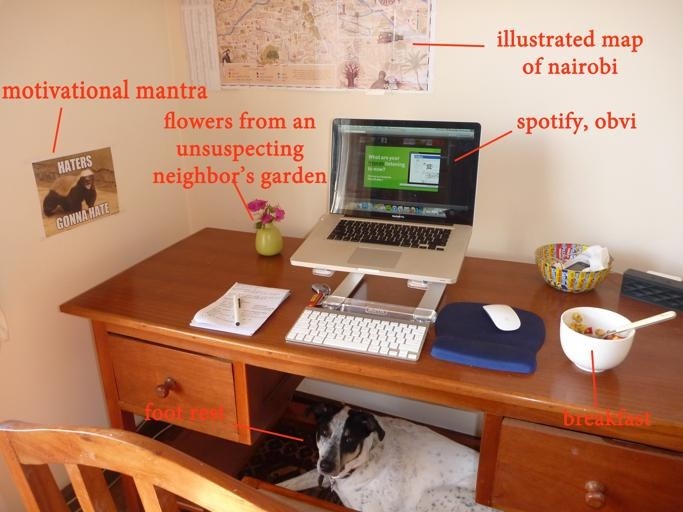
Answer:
255, 223, 282, 255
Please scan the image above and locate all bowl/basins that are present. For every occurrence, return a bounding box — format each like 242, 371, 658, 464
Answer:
559, 306, 636, 374
534, 243, 615, 294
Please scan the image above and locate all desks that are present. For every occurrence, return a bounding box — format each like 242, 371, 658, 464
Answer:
59, 227, 683, 512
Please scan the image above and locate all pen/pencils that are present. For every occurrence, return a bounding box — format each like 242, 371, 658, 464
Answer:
233, 294, 241, 326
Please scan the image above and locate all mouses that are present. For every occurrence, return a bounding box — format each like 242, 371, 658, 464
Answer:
482, 304, 522, 332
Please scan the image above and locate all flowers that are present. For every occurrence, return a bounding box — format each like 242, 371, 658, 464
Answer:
248, 199, 285, 229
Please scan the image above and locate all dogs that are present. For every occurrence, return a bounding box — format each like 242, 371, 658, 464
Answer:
275, 400, 500, 510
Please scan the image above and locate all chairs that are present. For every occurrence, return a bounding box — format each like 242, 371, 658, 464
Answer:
0, 420, 359, 512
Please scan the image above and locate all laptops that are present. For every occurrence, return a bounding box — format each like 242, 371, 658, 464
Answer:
289, 117, 482, 284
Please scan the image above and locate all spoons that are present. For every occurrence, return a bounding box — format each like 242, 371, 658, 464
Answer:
583, 310, 677, 340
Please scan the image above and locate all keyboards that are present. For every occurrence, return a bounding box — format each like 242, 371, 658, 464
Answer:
283, 306, 431, 365
